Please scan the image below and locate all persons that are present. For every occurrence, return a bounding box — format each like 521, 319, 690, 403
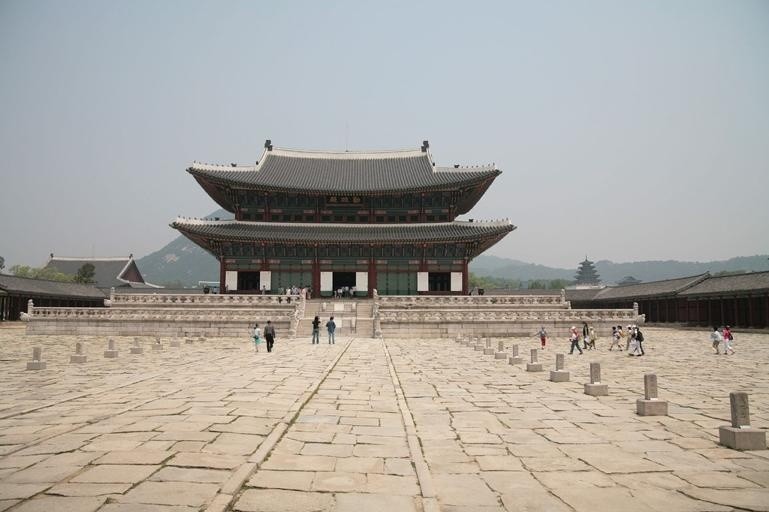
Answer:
533, 320, 645, 357
710, 325, 738, 355
252, 320, 276, 352
261, 285, 266, 294
277, 285, 357, 300
203, 284, 217, 294
311, 316, 336, 344
